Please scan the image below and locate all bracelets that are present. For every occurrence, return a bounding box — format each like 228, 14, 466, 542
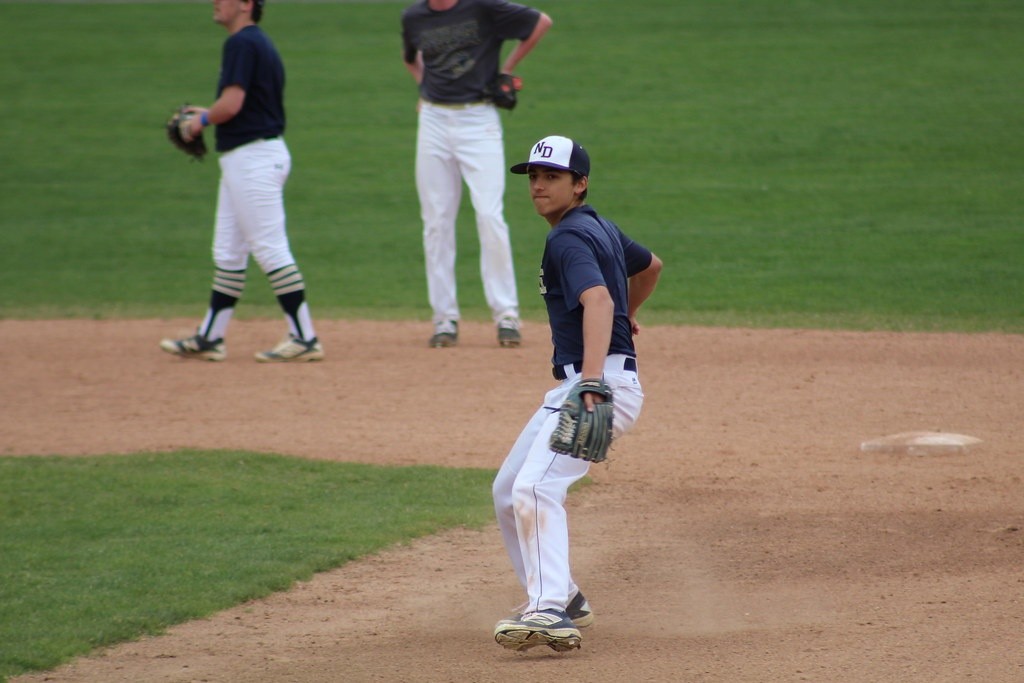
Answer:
200, 112, 210, 126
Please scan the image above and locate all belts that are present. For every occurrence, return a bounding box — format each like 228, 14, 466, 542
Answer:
428, 100, 482, 109
552, 357, 636, 381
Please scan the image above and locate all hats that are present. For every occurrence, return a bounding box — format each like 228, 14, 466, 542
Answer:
510, 136, 590, 178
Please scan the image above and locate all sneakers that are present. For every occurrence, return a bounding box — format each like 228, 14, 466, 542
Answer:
159, 334, 225, 361
430, 318, 457, 346
494, 608, 582, 652
496, 316, 520, 346
510, 590, 593, 627
253, 332, 324, 362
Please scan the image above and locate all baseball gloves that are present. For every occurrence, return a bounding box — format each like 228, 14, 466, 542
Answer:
164, 113, 208, 163
541, 377, 618, 472
491, 73, 523, 111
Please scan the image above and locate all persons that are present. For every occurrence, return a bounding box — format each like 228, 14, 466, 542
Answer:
160, 0, 324, 362
400, 0, 553, 348
492, 135, 663, 652
